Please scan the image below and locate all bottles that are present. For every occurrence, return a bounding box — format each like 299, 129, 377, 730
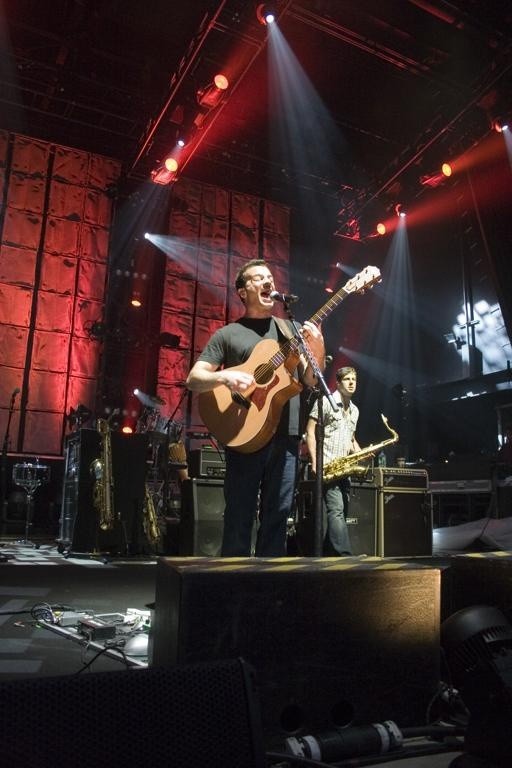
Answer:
378, 450, 387, 469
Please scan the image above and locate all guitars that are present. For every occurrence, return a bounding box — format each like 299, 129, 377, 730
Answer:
197, 264, 382, 453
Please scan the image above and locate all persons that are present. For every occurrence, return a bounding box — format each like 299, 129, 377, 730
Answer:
304, 363, 375, 557
186, 256, 327, 555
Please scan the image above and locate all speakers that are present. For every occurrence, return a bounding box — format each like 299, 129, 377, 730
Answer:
179, 478, 225, 557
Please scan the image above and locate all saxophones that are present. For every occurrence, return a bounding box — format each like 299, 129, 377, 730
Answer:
323, 414, 399, 483
91, 419, 115, 531
144, 490, 161, 545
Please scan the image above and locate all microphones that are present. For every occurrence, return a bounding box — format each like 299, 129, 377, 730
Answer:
269, 290, 299, 304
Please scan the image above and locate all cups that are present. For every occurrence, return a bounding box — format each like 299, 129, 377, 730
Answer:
396, 458, 405, 467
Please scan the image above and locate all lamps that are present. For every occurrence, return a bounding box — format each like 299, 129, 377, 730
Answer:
175, 116, 198, 149
126, 287, 144, 310
259, 5, 276, 25
492, 105, 509, 131
398, 196, 410, 219
